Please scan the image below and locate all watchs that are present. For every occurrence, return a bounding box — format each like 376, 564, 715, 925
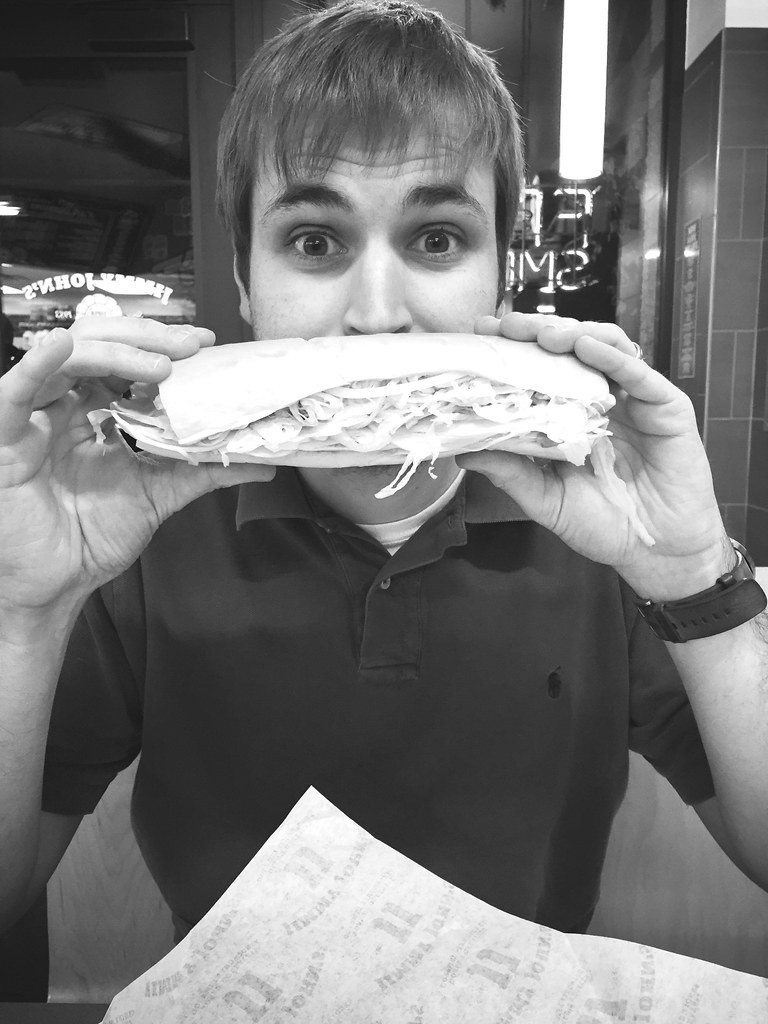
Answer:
633, 536, 768, 642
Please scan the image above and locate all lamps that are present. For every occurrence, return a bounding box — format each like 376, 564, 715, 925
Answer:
560, 1, 608, 181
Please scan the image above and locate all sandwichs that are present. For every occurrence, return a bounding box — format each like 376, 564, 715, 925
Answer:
84, 330, 655, 549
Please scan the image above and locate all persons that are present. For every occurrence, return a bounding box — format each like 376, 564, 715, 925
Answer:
0, 0, 768, 1005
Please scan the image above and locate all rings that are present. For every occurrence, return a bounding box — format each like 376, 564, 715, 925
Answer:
631, 342, 643, 360
